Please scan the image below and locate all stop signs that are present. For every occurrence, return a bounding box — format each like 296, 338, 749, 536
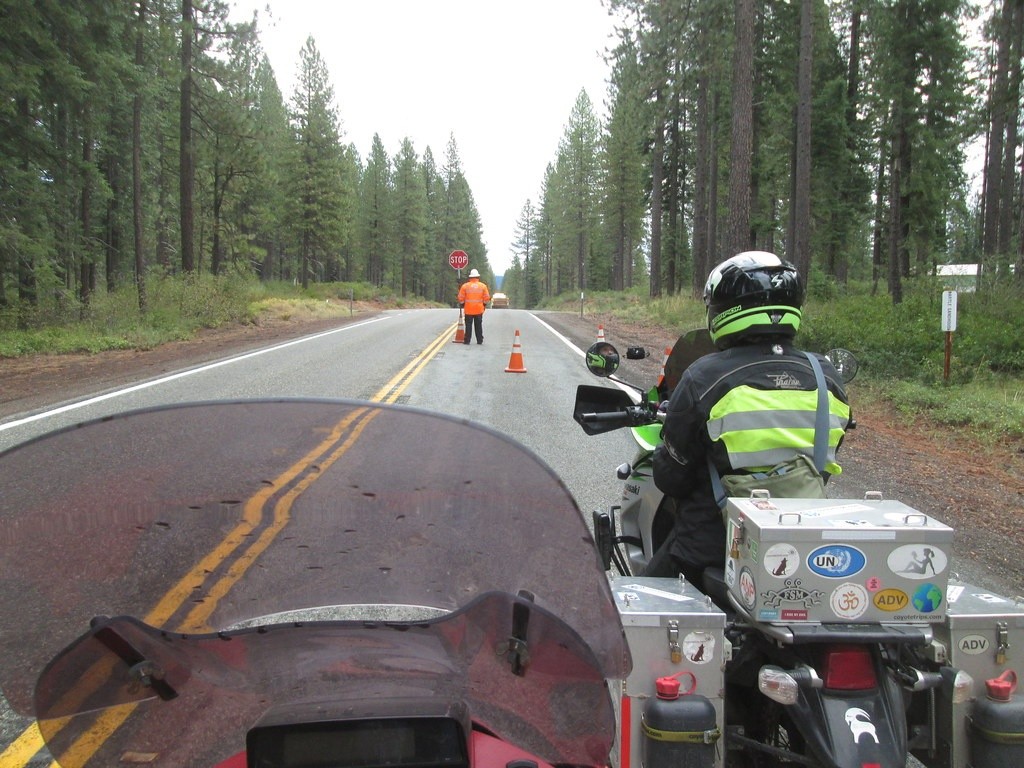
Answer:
449, 249, 469, 270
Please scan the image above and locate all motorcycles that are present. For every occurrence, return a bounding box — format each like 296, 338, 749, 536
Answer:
571, 340, 1024, 768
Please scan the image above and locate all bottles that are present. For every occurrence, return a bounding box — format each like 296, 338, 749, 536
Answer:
971, 669, 1024, 768
641, 672, 717, 768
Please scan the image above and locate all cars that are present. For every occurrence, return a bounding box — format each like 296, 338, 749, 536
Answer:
490, 292, 509, 309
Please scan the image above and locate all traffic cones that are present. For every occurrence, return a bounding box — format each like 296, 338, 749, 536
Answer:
594, 323, 606, 343
451, 312, 466, 343
504, 328, 528, 373
655, 345, 673, 385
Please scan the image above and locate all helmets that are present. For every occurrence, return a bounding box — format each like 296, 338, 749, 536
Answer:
702, 251, 802, 351
468, 269, 480, 278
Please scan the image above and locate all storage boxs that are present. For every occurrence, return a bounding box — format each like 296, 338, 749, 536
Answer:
725, 488, 953, 622
586, 572, 730, 768
944, 577, 1024, 767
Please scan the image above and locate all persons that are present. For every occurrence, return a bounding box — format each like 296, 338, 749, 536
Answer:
593, 342, 615, 358
641, 251, 855, 587
458, 268, 491, 345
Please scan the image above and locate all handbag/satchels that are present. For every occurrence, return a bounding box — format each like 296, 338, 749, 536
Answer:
718, 453, 828, 527
